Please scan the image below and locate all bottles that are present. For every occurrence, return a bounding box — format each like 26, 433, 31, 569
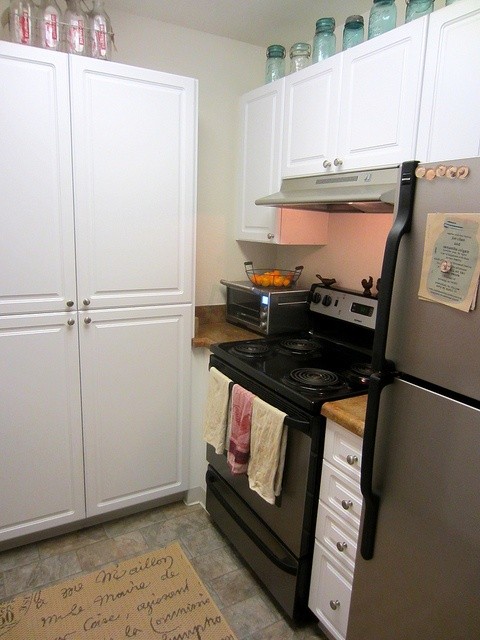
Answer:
8, 0, 37, 47
37, 0, 64, 51
342, 15, 364, 52
405, 0, 434, 25
367, 1, 396, 41
88, 0, 112, 61
312, 18, 336, 65
289, 43, 311, 74
264, 45, 286, 84
63, 0, 90, 56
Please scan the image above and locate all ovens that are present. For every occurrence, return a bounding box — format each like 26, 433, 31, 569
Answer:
205, 354, 327, 627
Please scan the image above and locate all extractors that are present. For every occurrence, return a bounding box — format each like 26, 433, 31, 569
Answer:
254, 163, 401, 214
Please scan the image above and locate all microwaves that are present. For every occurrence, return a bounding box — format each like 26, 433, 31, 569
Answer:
220, 279, 312, 335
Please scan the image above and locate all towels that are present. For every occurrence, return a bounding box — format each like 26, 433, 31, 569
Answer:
226, 384, 257, 474
247, 395, 288, 506
200, 365, 235, 455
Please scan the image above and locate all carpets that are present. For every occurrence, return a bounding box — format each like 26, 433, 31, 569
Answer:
0, 544, 239, 640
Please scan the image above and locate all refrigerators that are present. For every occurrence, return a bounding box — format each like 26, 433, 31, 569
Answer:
345, 155, 480, 640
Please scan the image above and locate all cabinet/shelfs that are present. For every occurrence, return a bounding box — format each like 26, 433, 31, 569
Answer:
283, 14, 430, 181
413, 1, 480, 164
307, 395, 369, 640
0, 41, 198, 552
235, 75, 328, 247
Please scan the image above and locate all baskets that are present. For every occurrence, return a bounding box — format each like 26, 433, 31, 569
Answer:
244, 262, 303, 289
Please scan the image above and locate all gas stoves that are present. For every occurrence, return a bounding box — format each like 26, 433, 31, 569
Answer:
208, 331, 373, 412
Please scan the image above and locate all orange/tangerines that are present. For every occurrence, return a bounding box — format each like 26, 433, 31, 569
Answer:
250, 270, 294, 287
